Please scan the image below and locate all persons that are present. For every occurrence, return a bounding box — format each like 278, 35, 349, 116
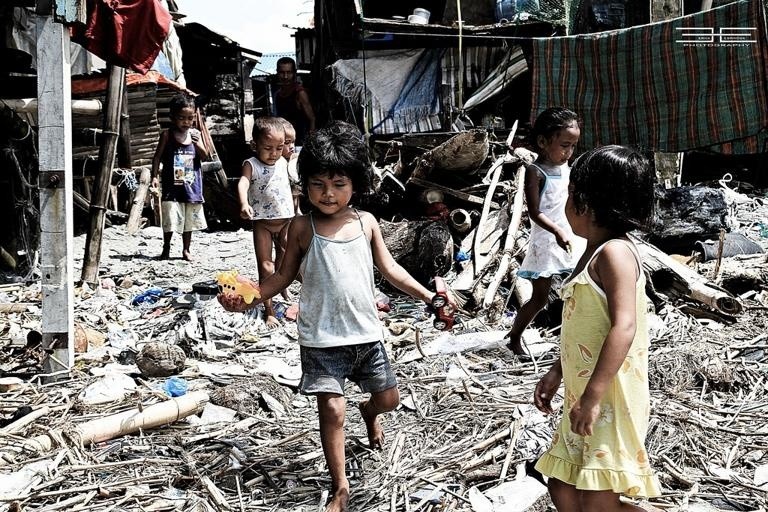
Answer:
533, 145, 661, 512
218, 123, 458, 512
267, 57, 316, 132
504, 107, 588, 363
274, 118, 304, 282
150, 94, 209, 262
238, 117, 294, 328
